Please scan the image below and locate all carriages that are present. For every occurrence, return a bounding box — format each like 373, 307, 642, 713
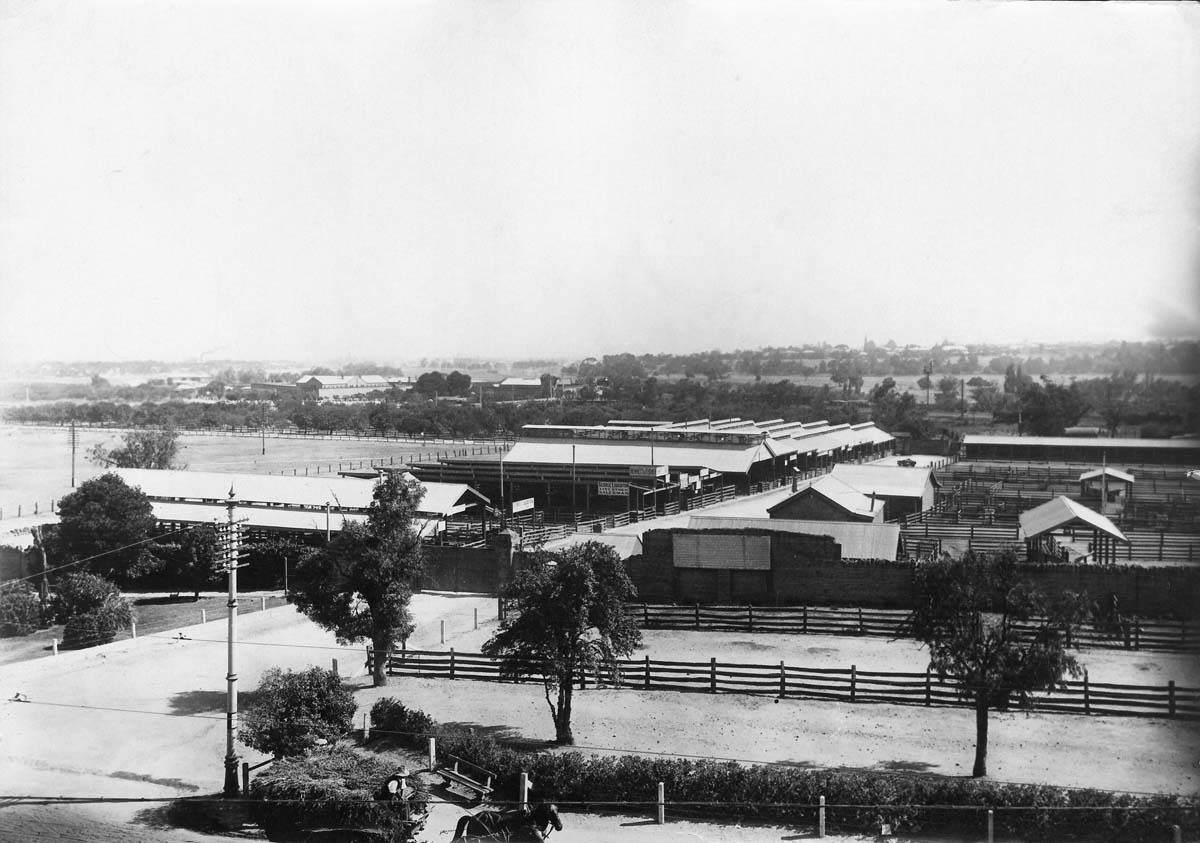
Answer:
243, 748, 562, 843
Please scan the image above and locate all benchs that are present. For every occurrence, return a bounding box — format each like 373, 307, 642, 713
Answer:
437, 754, 497, 804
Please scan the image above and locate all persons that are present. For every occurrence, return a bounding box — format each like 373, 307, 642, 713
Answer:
382, 764, 417, 828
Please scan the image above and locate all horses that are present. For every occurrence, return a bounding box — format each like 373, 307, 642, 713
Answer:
447, 801, 564, 843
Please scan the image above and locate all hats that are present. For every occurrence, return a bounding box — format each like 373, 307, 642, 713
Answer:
395, 765, 409, 776
